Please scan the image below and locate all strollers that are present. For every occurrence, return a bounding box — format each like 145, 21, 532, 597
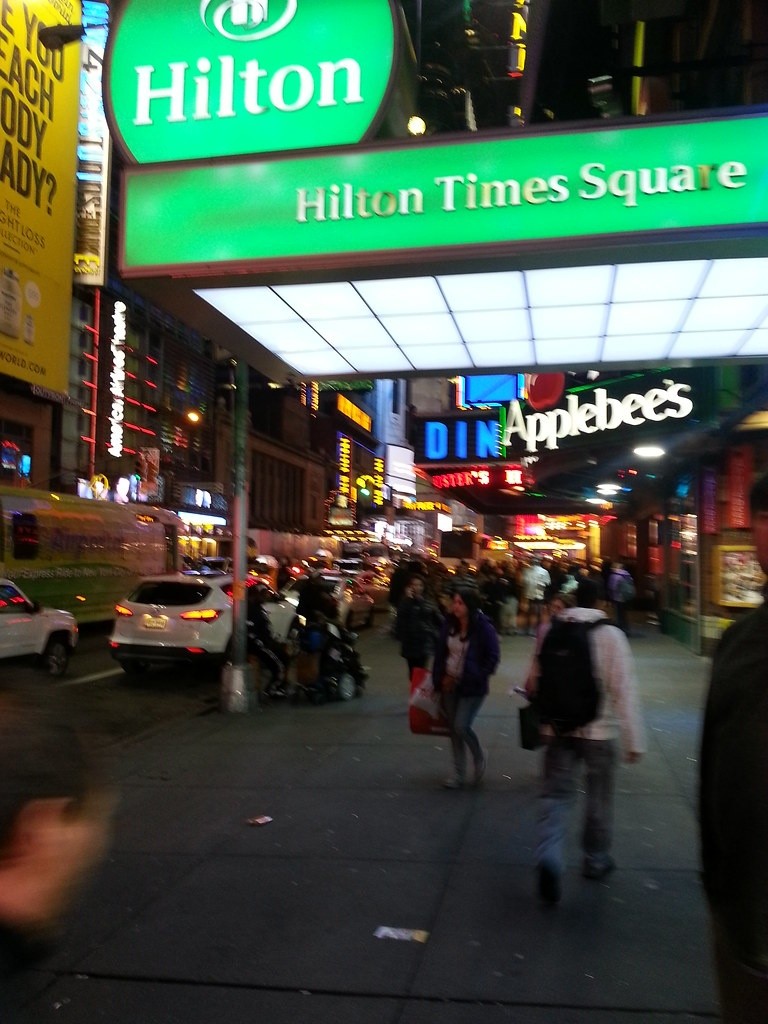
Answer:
296, 623, 368, 702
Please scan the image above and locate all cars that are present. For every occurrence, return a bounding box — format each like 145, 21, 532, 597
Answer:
280, 576, 377, 631
0, 578, 80, 678
108, 571, 285, 680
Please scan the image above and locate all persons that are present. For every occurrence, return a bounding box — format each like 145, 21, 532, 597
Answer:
247, 549, 637, 704
697, 472, 768, 1024
432, 588, 501, 788
0, 727, 117, 1024
525, 579, 644, 903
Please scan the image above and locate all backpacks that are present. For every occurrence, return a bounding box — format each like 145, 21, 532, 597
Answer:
539, 617, 619, 733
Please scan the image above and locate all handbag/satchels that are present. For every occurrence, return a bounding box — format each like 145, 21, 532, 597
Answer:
410, 666, 453, 737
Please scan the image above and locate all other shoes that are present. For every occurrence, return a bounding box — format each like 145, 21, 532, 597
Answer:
537, 860, 564, 907
446, 773, 471, 789
584, 859, 615, 880
474, 755, 488, 782
